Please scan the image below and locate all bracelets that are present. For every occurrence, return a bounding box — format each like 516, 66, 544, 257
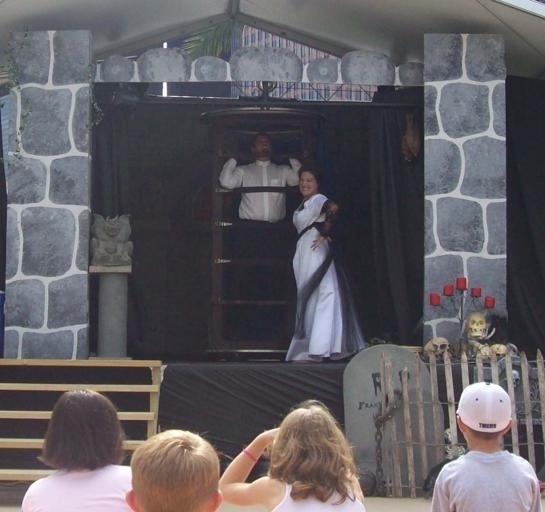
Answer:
243, 447, 259, 463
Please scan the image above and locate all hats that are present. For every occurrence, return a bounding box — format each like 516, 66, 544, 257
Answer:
455, 381, 512, 433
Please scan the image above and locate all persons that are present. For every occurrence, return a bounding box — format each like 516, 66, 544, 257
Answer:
18, 388, 135, 512
281, 161, 371, 364
428, 380, 545, 511
216, 399, 369, 511
123, 428, 224, 511
217, 130, 304, 307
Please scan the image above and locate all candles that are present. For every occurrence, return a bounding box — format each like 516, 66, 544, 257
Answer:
483, 295, 495, 309
456, 277, 466, 290
429, 293, 440, 305
443, 283, 455, 296
471, 287, 481, 297
